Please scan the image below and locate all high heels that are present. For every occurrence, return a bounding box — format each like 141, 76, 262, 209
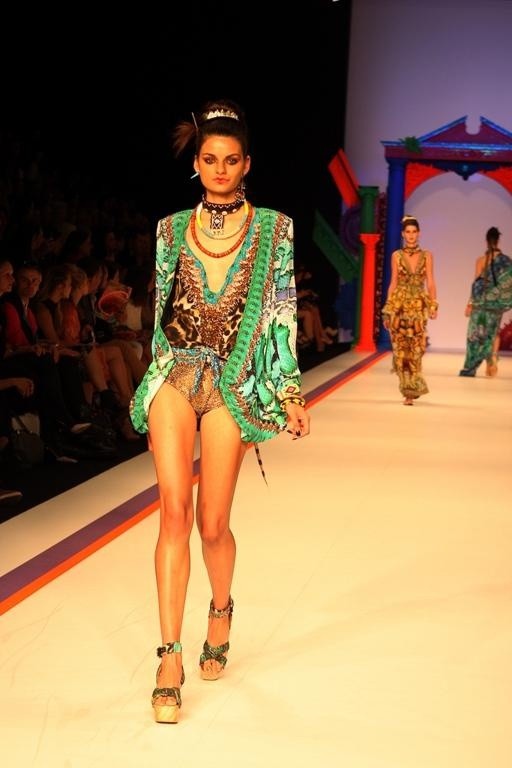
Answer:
100, 390, 129, 418
117, 417, 141, 444
487, 364, 498, 377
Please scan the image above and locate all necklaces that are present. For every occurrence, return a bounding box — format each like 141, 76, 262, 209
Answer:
200, 192, 245, 237
403, 244, 421, 253
190, 201, 256, 259
193, 203, 250, 241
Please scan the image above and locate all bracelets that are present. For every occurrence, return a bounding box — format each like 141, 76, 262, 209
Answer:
428, 300, 438, 310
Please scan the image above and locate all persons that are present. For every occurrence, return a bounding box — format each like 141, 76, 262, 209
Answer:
125, 100, 313, 727
3, 222, 154, 464
380, 214, 439, 409
293, 259, 336, 354
457, 227, 511, 379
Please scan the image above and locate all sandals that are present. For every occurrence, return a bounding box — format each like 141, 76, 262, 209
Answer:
404, 395, 412, 407
302, 328, 338, 356
151, 643, 185, 725
200, 597, 233, 681
